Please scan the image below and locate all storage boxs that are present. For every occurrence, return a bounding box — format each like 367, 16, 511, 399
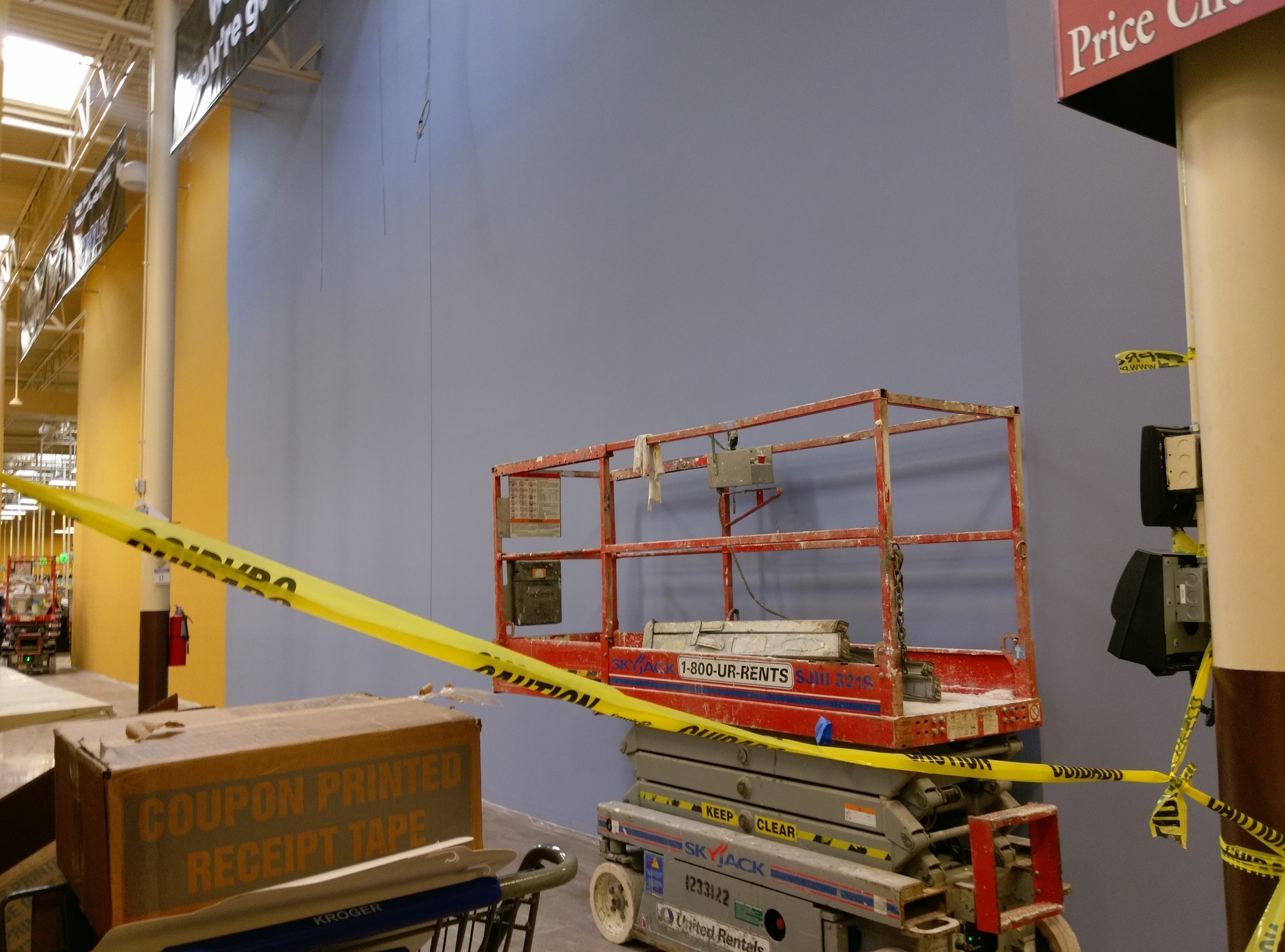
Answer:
52, 692, 483, 935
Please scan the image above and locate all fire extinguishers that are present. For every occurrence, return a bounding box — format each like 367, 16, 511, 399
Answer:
169, 604, 193, 667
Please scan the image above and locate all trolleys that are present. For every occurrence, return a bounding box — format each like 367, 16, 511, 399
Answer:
0, 842, 579, 952
